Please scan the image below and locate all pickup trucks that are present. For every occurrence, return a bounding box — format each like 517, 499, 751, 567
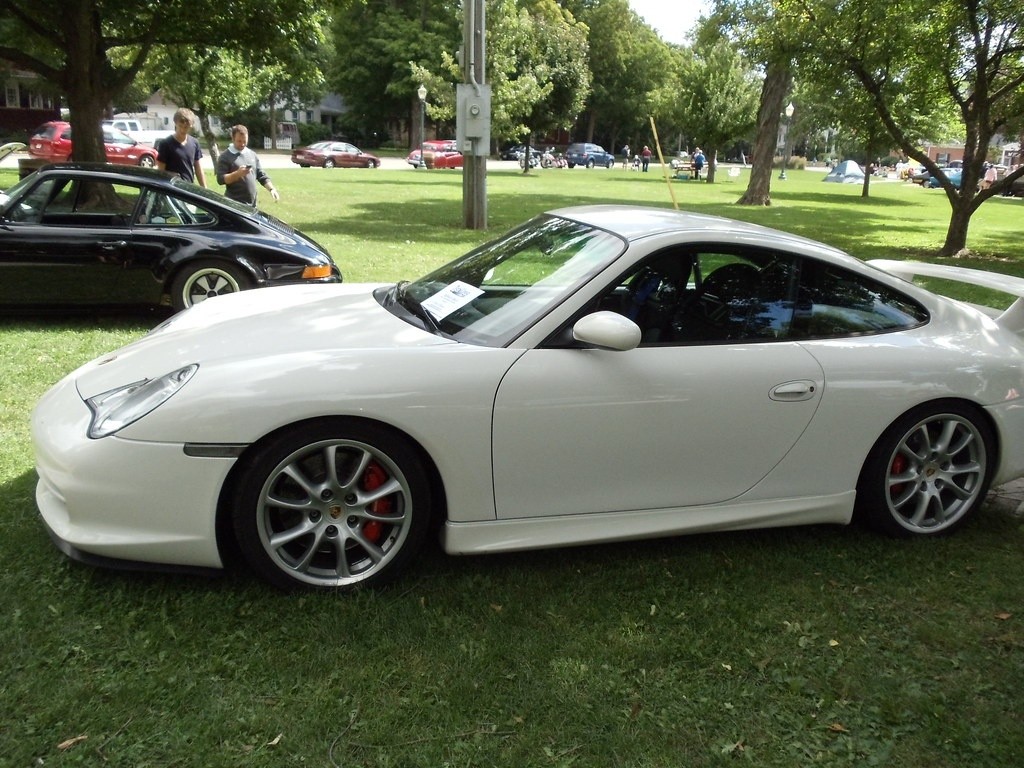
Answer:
105, 119, 174, 150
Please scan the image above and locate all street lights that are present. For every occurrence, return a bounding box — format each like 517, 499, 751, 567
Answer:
417, 84, 428, 169
778, 101, 795, 179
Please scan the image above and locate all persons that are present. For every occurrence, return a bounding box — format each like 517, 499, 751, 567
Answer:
695, 151, 705, 167
642, 146, 651, 172
874, 160, 903, 179
979, 162, 1013, 196
155, 108, 208, 188
691, 148, 699, 161
217, 125, 278, 208
621, 145, 629, 168
633, 155, 641, 171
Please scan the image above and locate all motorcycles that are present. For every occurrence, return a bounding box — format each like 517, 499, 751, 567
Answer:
540, 147, 567, 169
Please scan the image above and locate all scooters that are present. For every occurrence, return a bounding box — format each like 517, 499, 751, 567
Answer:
631, 161, 640, 173
516, 149, 538, 168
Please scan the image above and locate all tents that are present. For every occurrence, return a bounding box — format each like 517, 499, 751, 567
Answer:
822, 160, 865, 184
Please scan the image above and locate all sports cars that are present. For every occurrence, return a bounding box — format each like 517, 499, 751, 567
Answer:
29, 208, 1024, 598
0, 162, 344, 331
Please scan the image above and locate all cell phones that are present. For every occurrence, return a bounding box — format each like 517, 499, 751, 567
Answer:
246, 165, 252, 169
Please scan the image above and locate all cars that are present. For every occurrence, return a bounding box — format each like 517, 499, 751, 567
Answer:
291, 141, 381, 169
990, 164, 1008, 181
405, 140, 464, 169
677, 151, 688, 158
499, 143, 541, 161
929, 160, 964, 190
913, 164, 946, 189
29, 119, 162, 171
1000, 169, 1024, 198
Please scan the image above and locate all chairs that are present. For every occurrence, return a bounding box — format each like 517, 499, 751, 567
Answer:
615, 253, 692, 345
679, 264, 760, 346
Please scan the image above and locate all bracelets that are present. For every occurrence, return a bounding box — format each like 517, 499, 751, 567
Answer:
270, 188, 274, 192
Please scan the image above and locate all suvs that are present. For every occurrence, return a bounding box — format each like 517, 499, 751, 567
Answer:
566, 142, 615, 170
